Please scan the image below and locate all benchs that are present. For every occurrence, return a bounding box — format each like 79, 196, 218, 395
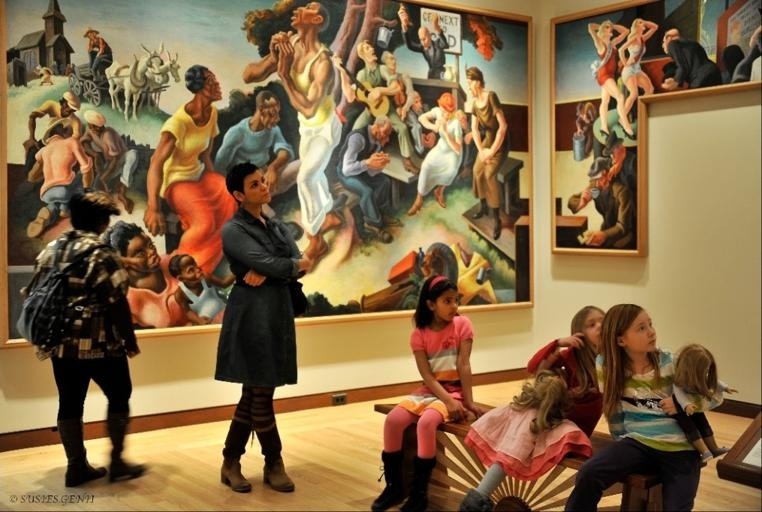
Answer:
375, 398, 707, 512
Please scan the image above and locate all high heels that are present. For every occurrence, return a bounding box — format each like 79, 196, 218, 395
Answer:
493, 219, 501, 240
600, 119, 636, 141
473, 206, 490, 218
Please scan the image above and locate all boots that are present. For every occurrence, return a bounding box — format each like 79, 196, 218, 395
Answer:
58, 414, 107, 486
371, 451, 406, 512
400, 456, 436, 511
106, 415, 145, 482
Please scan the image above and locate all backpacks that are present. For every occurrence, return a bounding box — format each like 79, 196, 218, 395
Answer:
15, 239, 105, 350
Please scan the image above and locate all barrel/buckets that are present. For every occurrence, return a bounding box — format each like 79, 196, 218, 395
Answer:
375, 22, 394, 48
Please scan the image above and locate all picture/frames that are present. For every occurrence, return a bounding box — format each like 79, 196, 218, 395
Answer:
1, 0, 536, 350
549, 0, 762, 255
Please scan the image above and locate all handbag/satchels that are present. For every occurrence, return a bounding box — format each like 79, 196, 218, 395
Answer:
288, 280, 314, 316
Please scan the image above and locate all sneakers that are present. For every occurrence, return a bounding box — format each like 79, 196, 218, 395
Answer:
460, 488, 494, 512
407, 200, 423, 216
364, 215, 400, 243
26, 207, 52, 238
434, 191, 446, 208
403, 160, 420, 174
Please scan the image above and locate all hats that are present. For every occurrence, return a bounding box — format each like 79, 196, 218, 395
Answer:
588, 157, 611, 179
84, 111, 106, 126
82, 26, 99, 37
63, 91, 81, 111
436, 93, 458, 112
67, 192, 121, 216
41, 117, 72, 144
602, 131, 624, 156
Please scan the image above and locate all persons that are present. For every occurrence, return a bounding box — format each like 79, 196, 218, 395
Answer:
34, 191, 146, 487
672, 345, 738, 464
215, 162, 311, 492
371, 276, 485, 512
27, 1, 507, 328
463, 371, 593, 480
564, 304, 723, 512
459, 306, 606, 512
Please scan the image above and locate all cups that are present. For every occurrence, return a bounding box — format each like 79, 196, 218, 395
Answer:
476, 267, 487, 284
590, 189, 601, 200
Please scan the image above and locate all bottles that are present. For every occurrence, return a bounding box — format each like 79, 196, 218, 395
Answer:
399, 2, 414, 29
455, 242, 471, 269
417, 246, 425, 269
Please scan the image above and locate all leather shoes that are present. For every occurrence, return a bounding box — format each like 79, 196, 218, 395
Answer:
264, 460, 296, 492
221, 460, 253, 492
